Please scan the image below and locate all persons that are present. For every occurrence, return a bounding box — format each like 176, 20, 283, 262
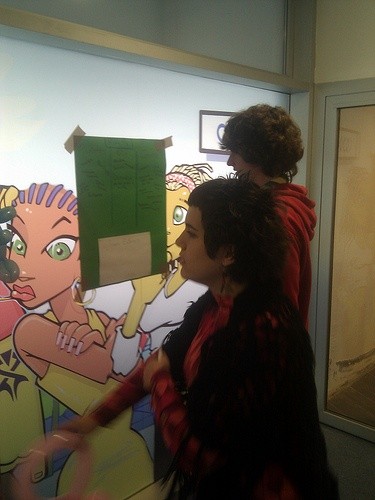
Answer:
58, 168, 341, 500
214, 103, 318, 333
